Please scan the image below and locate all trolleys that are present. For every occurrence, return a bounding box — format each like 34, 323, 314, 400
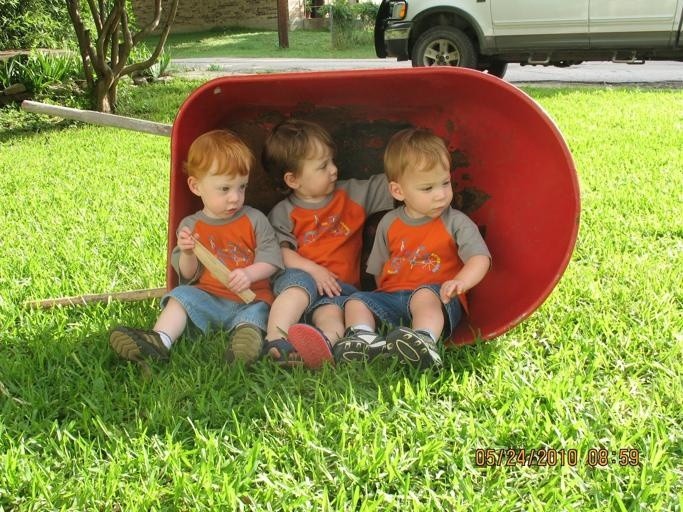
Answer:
19, 64, 581, 353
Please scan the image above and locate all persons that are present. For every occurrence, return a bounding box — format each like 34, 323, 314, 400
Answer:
332, 126, 493, 376
261, 120, 395, 374
108, 129, 285, 379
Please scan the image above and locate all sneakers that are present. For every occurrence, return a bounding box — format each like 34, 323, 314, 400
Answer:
230, 323, 265, 365
109, 327, 170, 363
260, 337, 302, 366
333, 331, 394, 364
288, 323, 335, 370
386, 326, 443, 375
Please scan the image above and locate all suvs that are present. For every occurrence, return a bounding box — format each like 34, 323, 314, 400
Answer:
372, 0, 682, 79
